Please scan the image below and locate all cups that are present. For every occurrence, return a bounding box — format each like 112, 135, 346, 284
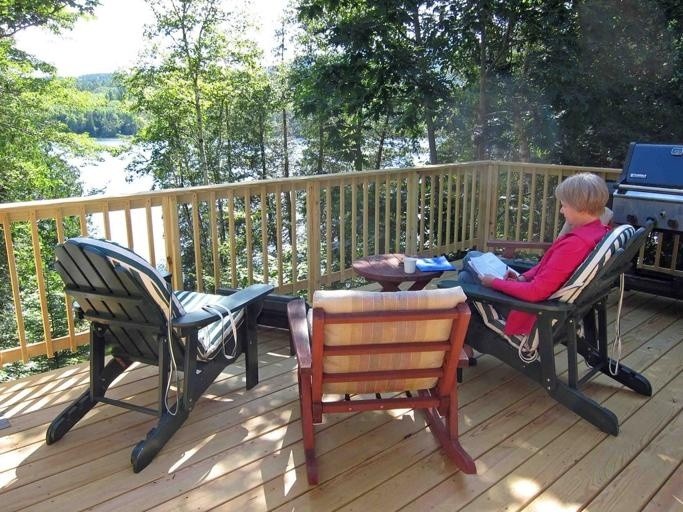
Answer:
403, 258, 417, 274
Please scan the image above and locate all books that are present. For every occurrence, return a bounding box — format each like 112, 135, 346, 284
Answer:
412, 254, 456, 272
468, 250, 521, 280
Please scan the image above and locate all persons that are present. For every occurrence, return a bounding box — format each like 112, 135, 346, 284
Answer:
457, 168, 613, 366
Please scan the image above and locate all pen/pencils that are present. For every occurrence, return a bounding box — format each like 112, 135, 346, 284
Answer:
505, 266, 509, 278
